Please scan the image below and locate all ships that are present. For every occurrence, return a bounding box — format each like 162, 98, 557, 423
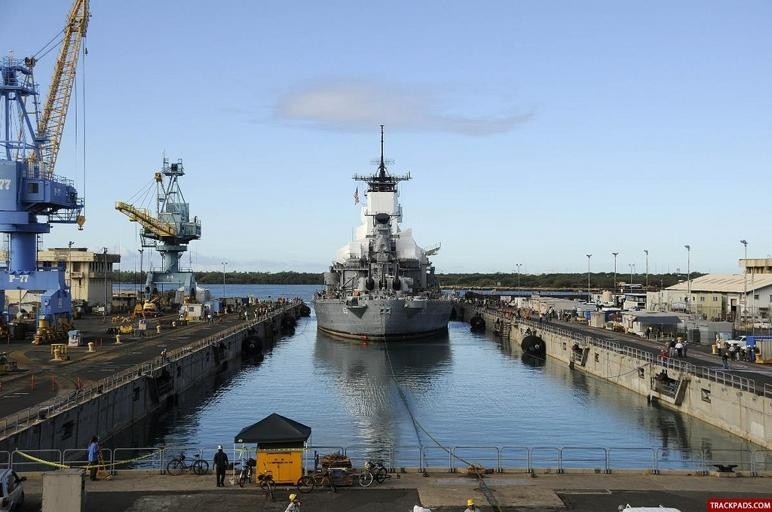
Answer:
313, 123, 454, 343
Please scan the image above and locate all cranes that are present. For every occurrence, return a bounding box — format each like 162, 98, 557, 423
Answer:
0, 0, 93, 346
114, 155, 202, 306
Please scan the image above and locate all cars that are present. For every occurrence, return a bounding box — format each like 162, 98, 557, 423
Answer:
0, 468, 27, 512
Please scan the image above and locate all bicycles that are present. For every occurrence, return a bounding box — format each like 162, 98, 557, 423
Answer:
257, 470, 275, 501
295, 454, 388, 494
239, 457, 256, 488
166, 453, 209, 476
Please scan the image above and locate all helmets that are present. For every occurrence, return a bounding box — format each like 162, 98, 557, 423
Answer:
467, 499, 475, 506
289, 493, 297, 501
217, 445, 224, 450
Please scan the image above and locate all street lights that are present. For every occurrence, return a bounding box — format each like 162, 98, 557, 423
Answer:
740, 239, 749, 336
644, 249, 649, 286
684, 245, 691, 293
612, 252, 619, 288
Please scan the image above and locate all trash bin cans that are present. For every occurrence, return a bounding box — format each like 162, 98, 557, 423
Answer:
41, 469, 86, 512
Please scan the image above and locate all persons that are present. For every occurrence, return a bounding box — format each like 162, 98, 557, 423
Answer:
464, 498, 481, 512
285, 494, 304, 512
646, 327, 650, 339
212, 444, 229, 487
207, 302, 274, 324
717, 341, 759, 369
495, 299, 554, 321
668, 339, 687, 358
88, 436, 101, 479
